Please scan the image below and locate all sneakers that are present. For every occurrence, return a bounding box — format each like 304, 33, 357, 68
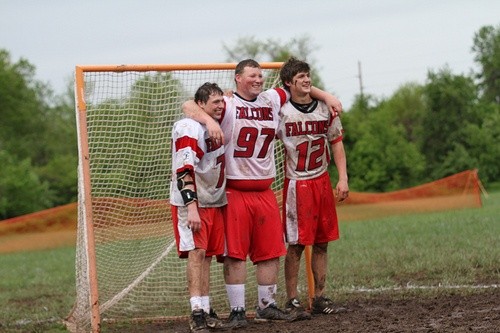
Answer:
311, 296, 341, 314
228, 307, 250, 330
254, 305, 297, 322
202, 308, 223, 329
285, 297, 311, 320
190, 308, 209, 333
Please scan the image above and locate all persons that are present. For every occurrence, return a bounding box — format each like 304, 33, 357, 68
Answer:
169, 82, 229, 333
181, 58, 343, 330
220, 55, 350, 320
